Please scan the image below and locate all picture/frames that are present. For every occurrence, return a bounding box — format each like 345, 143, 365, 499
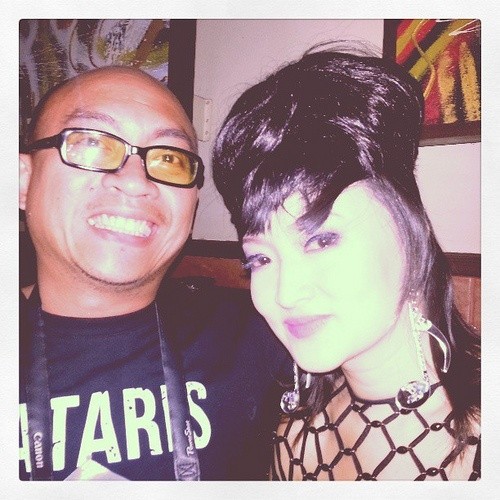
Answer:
19, 19, 197, 157
382, 19, 482, 148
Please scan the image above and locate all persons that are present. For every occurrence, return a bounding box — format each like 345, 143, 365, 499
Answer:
210, 36, 481, 481
18, 64, 302, 482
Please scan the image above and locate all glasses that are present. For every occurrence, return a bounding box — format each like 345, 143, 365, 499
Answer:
20, 126, 206, 190
